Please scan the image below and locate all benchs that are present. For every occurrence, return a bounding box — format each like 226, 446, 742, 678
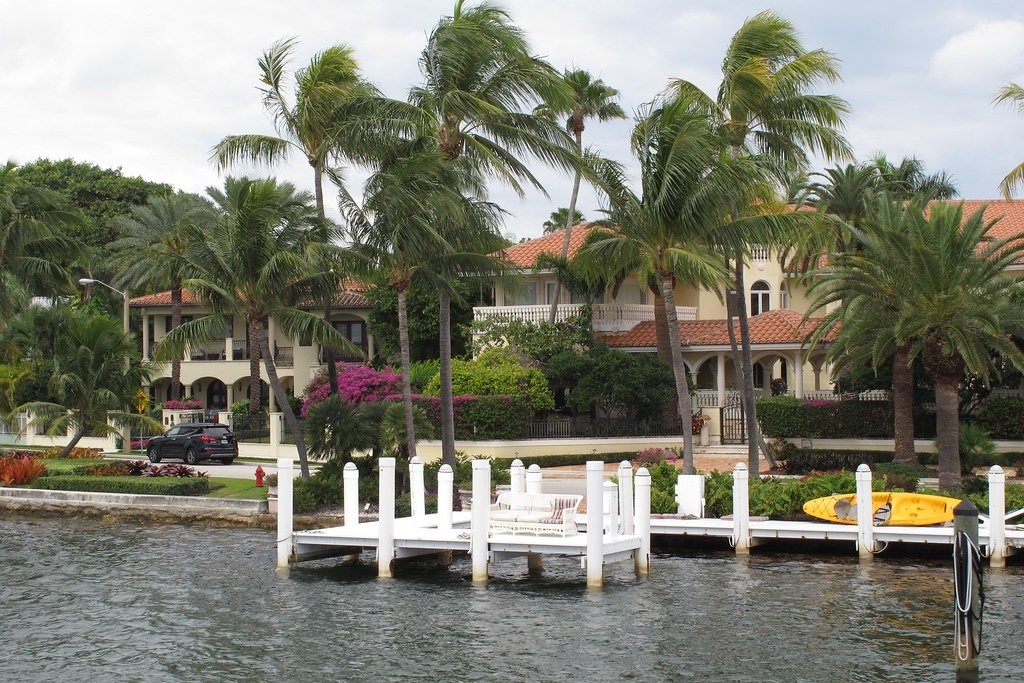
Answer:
488, 492, 584, 537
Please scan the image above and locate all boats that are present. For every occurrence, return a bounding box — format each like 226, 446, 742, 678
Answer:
802, 491, 962, 527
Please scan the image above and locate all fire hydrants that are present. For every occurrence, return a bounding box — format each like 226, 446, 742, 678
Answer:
254, 465, 265, 486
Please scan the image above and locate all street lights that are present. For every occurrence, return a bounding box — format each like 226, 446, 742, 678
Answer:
79, 279, 131, 454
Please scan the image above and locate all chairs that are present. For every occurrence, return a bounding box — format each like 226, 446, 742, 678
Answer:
222, 347, 243, 360
190, 347, 206, 360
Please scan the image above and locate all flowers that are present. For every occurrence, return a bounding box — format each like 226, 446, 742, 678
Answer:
163, 400, 203, 411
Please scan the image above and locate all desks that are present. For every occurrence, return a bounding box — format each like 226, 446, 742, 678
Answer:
208, 354, 219, 360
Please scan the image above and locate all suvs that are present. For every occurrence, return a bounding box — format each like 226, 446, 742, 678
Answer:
146, 423, 238, 465
